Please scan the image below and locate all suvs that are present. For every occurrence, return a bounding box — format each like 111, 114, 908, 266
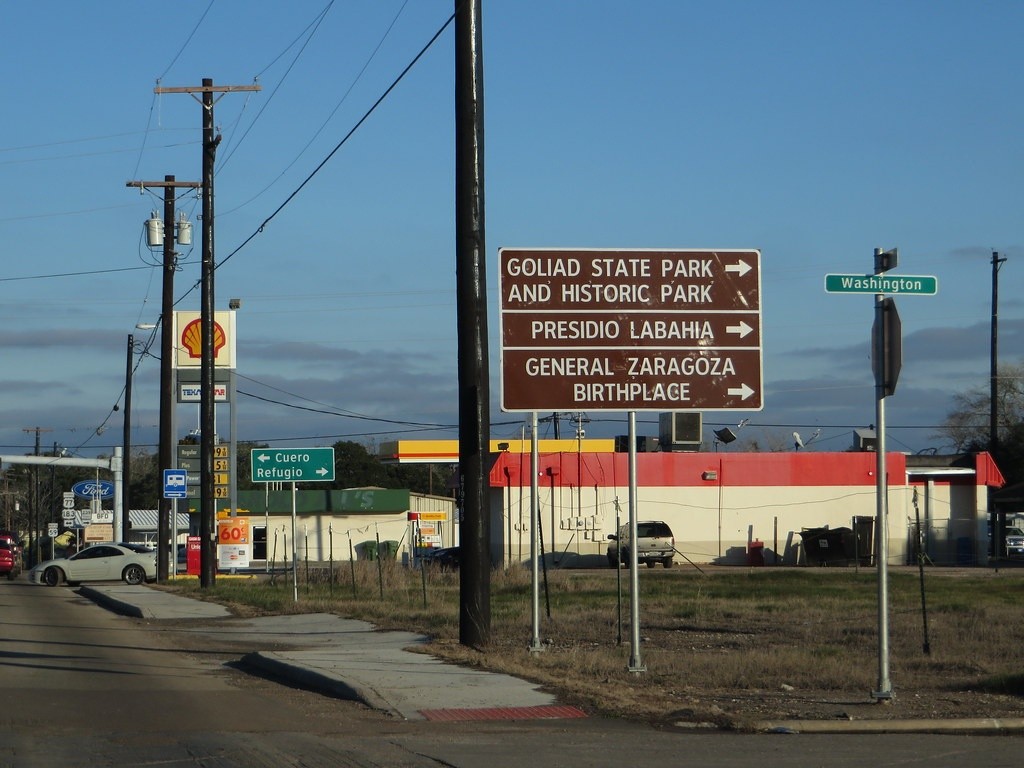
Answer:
607, 520, 676, 568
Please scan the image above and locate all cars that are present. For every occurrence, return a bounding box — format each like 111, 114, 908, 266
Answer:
29, 542, 174, 585
0, 531, 23, 578
1004, 526, 1024, 557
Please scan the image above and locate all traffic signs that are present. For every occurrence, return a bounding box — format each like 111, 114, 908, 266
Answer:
498, 246, 763, 312
251, 448, 336, 483
498, 347, 765, 413
500, 311, 764, 347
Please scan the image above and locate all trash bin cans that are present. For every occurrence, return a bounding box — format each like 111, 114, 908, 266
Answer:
384, 541, 399, 562
365, 541, 377, 561
798, 529, 862, 568
